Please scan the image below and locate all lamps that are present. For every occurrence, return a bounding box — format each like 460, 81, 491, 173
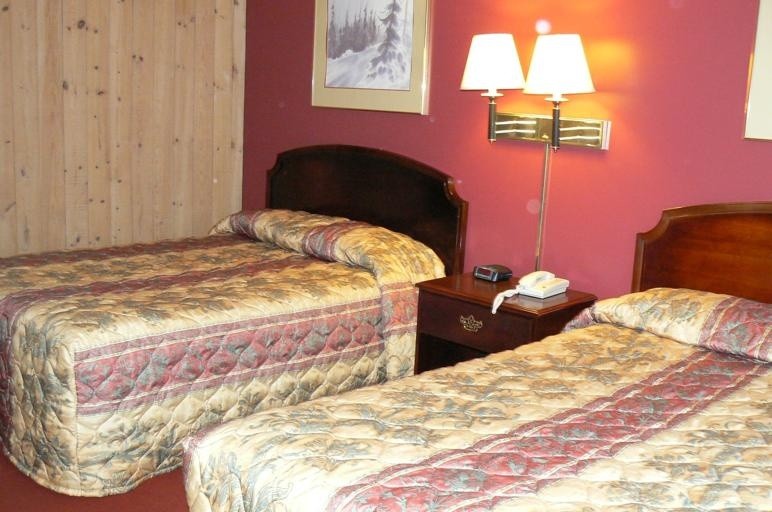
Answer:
312, 0, 433, 115
459, 33, 611, 153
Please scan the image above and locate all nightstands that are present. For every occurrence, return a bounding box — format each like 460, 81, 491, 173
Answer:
415, 271, 597, 374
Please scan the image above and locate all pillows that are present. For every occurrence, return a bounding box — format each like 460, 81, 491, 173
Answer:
210, 209, 446, 289
565, 288, 772, 366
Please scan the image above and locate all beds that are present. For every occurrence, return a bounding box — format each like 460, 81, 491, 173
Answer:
0, 144, 469, 498
182, 202, 772, 512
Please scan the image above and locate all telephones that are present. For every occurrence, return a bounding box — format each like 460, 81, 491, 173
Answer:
515, 271, 570, 299
